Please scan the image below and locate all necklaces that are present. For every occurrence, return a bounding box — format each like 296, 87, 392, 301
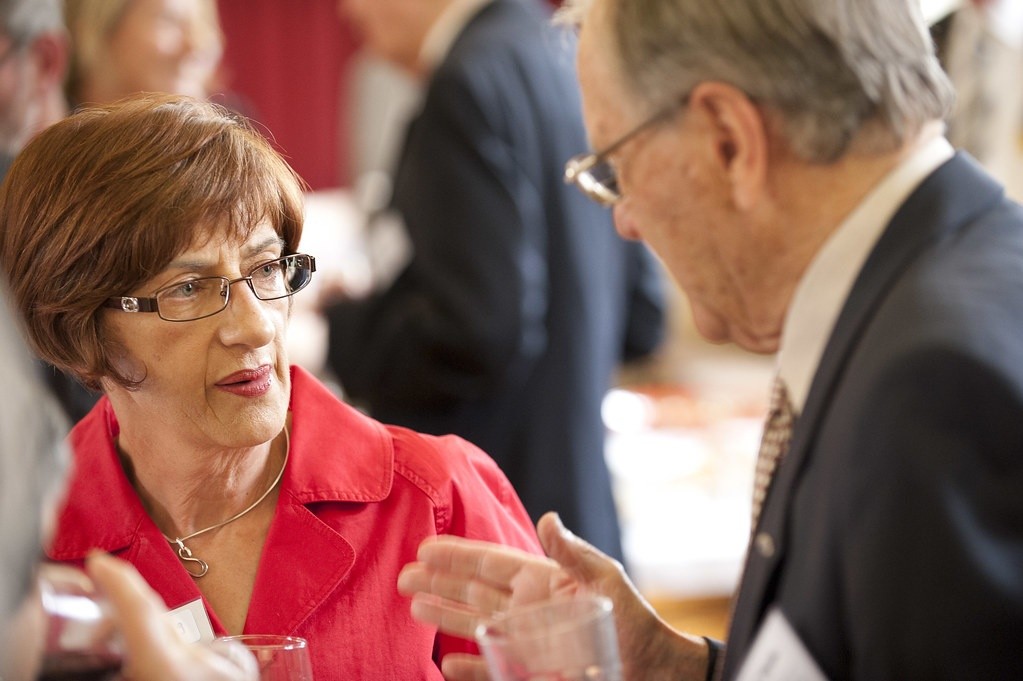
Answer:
161, 425, 290, 578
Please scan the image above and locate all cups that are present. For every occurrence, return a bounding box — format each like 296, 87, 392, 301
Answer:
218, 634, 313, 681
474, 594, 623, 681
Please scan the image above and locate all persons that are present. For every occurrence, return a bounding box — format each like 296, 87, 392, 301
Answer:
293, 1, 668, 571
0, 96, 548, 680
0, 0, 223, 149
397, 1, 1023, 681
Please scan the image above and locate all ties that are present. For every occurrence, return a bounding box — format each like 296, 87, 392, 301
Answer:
746, 380, 796, 536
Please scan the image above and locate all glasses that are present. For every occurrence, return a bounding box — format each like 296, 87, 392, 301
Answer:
562, 85, 762, 207
101, 252, 316, 322
